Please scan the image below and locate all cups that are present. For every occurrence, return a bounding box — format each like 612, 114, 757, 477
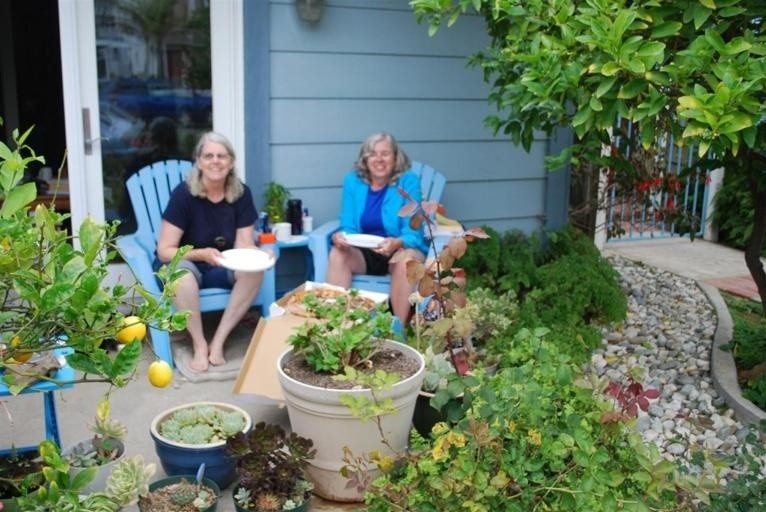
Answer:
272, 222, 292, 241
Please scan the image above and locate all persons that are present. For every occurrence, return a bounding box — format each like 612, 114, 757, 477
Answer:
155, 131, 275, 372
325, 132, 435, 345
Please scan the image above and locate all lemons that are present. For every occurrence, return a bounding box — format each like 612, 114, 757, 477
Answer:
11, 336, 33, 363
148, 360, 172, 387
115, 316, 146, 344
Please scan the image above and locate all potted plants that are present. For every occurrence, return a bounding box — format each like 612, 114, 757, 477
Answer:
277, 282, 520, 503
0, 400, 321, 512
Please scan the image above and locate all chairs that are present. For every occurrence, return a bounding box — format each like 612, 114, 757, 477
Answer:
115, 160, 278, 368
307, 159, 464, 292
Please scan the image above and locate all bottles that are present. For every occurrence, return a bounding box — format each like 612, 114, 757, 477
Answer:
286, 199, 302, 234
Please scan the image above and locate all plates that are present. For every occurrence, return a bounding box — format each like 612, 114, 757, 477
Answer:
215, 248, 273, 271
344, 233, 386, 248
